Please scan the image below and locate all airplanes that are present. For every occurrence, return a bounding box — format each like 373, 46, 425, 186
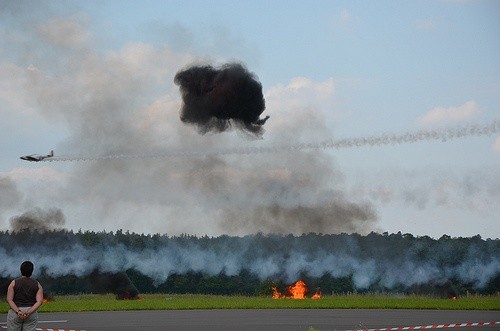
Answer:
20, 150, 54, 162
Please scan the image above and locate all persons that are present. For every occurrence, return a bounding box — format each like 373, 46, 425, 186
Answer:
6, 261, 44, 331
